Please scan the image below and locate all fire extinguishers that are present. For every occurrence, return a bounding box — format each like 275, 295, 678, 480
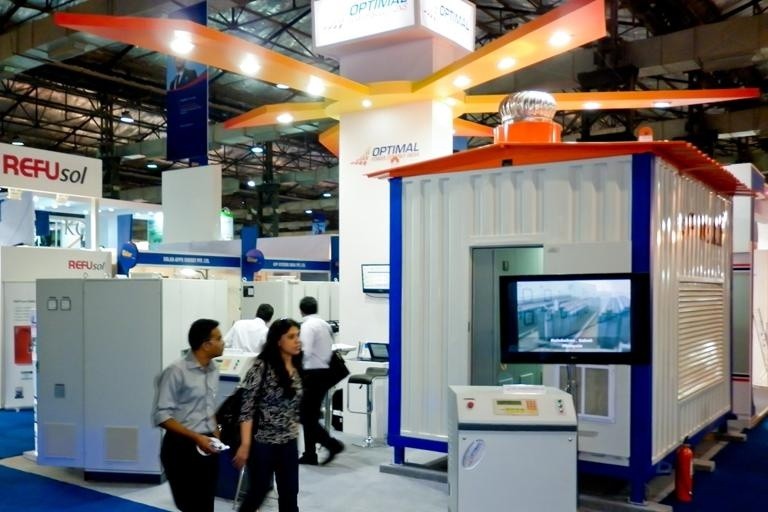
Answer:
675, 436, 693, 503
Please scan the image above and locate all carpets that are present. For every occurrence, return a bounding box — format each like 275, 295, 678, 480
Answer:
0, 408, 179, 512
660, 413, 767, 512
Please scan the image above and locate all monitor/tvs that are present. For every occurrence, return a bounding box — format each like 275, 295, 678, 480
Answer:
499, 272, 652, 366
360, 263, 389, 292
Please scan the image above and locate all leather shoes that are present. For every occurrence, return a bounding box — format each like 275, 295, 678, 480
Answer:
298, 444, 343, 466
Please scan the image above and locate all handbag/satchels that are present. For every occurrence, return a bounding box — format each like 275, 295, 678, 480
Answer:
216, 387, 259, 448
328, 351, 349, 390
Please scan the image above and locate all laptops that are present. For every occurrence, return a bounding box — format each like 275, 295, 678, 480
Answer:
368, 343, 390, 361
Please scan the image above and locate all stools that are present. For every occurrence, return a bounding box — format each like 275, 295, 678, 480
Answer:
347, 367, 388, 448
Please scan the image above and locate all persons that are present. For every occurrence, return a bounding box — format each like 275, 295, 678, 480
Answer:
229, 317, 306, 512
294, 295, 346, 467
150, 319, 231, 512
223, 302, 276, 354
